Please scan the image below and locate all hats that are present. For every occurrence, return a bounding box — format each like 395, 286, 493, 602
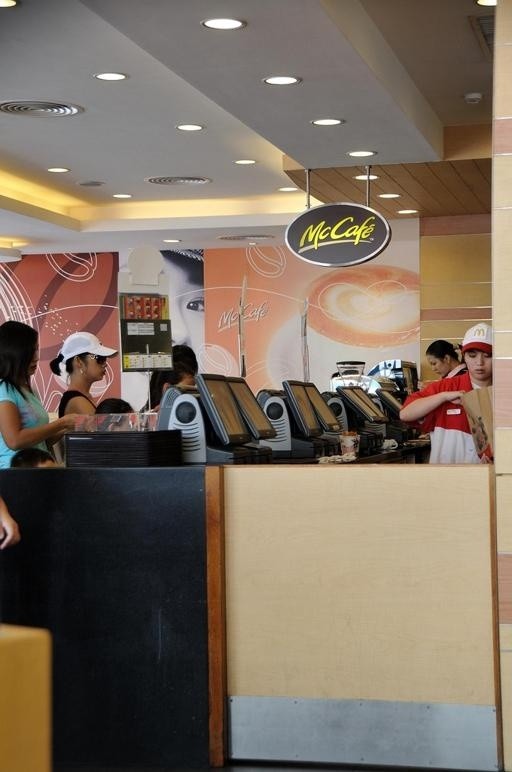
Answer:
460, 322, 493, 355
56, 331, 120, 366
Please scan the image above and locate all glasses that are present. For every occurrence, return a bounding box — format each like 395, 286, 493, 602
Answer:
84, 354, 106, 365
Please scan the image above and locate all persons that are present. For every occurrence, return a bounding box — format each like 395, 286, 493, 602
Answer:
95, 397, 136, 415
0, 495, 23, 552
0, 320, 92, 470
417, 339, 469, 392
141, 343, 200, 403
49, 331, 119, 429
398, 319, 493, 463
153, 248, 204, 357
11, 447, 62, 468
141, 361, 196, 425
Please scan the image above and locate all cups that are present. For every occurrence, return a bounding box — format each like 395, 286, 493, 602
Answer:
339, 432, 361, 462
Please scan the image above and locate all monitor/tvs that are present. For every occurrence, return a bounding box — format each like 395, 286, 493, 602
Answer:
195, 374, 252, 447
336, 386, 382, 423
375, 389, 403, 416
282, 380, 323, 437
304, 382, 343, 432
226, 377, 277, 440
349, 386, 389, 423
